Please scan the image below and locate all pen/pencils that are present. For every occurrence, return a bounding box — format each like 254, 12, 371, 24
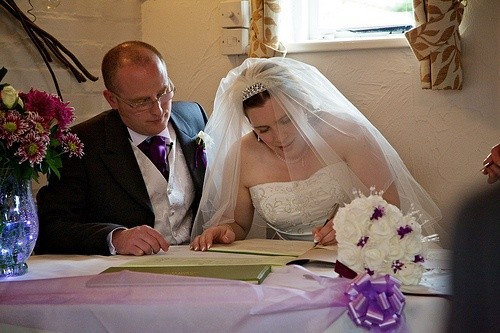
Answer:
311, 202, 337, 249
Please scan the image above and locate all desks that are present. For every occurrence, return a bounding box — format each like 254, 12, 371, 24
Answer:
0, 236, 449, 333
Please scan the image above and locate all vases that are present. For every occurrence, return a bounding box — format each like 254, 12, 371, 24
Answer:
0, 164, 39, 278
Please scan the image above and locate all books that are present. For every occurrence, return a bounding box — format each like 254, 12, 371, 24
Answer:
85, 264, 271, 287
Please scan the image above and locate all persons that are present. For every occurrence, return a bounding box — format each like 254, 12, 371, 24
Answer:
480, 144, 500, 185
190, 57, 443, 252
33, 41, 208, 256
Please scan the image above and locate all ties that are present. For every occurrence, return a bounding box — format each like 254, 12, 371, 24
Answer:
139, 135, 170, 176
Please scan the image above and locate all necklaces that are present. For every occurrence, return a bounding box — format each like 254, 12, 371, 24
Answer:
276, 147, 310, 164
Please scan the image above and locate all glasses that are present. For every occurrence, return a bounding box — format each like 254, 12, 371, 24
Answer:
110, 80, 176, 112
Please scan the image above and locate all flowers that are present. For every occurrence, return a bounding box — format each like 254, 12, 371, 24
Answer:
331, 193, 426, 287
196, 130, 215, 154
0, 80, 88, 182
